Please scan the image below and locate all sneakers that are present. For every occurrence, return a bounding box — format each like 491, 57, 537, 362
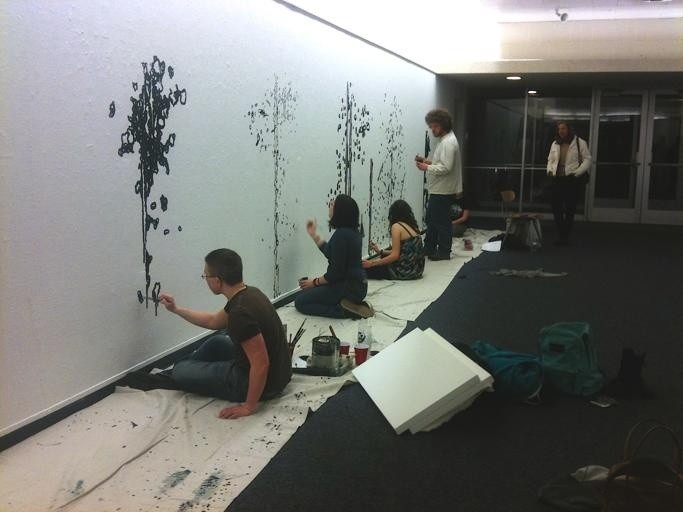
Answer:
340, 298, 374, 317
423, 247, 450, 260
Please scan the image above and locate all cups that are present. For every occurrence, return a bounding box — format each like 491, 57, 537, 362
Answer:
353, 343, 369, 366
339, 343, 350, 357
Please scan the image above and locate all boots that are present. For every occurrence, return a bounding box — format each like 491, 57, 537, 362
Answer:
607, 347, 646, 396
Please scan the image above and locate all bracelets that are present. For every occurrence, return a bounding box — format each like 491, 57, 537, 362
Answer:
317, 279, 321, 286
312, 278, 318, 287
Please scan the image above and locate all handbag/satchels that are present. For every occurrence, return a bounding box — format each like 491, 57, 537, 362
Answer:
469, 340, 544, 404
501, 213, 544, 251
538, 416, 682, 512
577, 172, 589, 184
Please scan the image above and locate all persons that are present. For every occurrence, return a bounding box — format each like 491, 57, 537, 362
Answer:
412, 107, 463, 262
445, 189, 470, 237
292, 193, 376, 320
120, 247, 293, 420
359, 200, 426, 282
545, 120, 593, 246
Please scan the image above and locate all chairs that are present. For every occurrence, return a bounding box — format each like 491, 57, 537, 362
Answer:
501, 190, 544, 249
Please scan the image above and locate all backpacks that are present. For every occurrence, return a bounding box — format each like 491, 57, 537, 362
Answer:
534, 321, 605, 398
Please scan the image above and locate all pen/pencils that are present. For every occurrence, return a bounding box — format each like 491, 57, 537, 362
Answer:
140, 297, 172, 302
284, 318, 307, 346
